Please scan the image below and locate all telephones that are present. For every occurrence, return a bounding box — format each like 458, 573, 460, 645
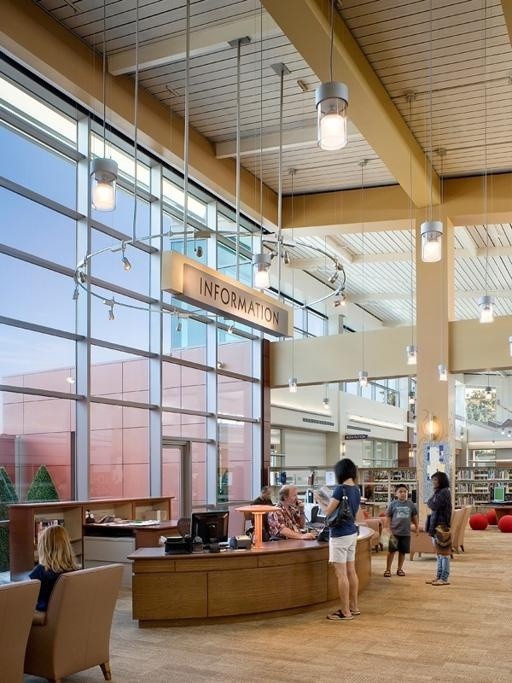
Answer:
164, 534, 193, 554
295, 501, 298, 506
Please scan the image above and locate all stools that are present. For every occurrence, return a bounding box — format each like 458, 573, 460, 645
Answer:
469, 510, 512, 532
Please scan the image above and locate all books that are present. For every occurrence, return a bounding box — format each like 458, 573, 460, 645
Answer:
34, 517, 65, 544
364, 467, 415, 502
455, 467, 512, 505
311, 484, 333, 506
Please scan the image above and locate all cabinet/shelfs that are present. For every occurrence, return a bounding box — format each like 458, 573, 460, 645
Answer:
356, 467, 418, 506
7, 494, 177, 583
454, 466, 512, 508
268, 465, 360, 505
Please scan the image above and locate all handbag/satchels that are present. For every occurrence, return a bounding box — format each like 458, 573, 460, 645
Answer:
425, 514, 432, 533
324, 484, 353, 528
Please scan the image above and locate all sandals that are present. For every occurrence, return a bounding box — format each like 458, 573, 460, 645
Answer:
384, 570, 391, 577
431, 581, 451, 586
327, 610, 354, 621
349, 610, 361, 615
425, 579, 437, 584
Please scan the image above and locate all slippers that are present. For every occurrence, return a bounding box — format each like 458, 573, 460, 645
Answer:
397, 569, 405, 576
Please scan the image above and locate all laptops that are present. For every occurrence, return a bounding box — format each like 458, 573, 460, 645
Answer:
491, 487, 505, 502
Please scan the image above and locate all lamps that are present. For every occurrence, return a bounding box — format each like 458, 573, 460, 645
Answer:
251, 4, 272, 289
408, 391, 416, 405
419, 1, 444, 263
289, 173, 298, 393
74, 230, 347, 330
485, 371, 492, 400
341, 441, 346, 456
89, 1, 118, 212
322, 396, 331, 409
358, 166, 369, 387
315, 1, 349, 151
477, 0, 495, 324
438, 153, 448, 382
428, 412, 436, 441
406, 99, 418, 365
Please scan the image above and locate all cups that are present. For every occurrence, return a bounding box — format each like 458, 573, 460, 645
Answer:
208, 538, 220, 553
191, 536, 203, 554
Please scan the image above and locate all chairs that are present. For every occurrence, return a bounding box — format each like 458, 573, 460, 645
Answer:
409, 505, 474, 562
1, 579, 43, 681
355, 508, 384, 553
262, 514, 283, 542
23, 562, 125, 682
177, 518, 191, 536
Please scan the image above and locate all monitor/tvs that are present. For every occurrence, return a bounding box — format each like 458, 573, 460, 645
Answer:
191, 510, 229, 550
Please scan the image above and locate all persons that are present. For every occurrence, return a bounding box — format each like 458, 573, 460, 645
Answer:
425, 473, 453, 586
28, 525, 82, 613
312, 458, 360, 620
266, 484, 317, 540
250, 486, 274, 529
383, 484, 419, 577
